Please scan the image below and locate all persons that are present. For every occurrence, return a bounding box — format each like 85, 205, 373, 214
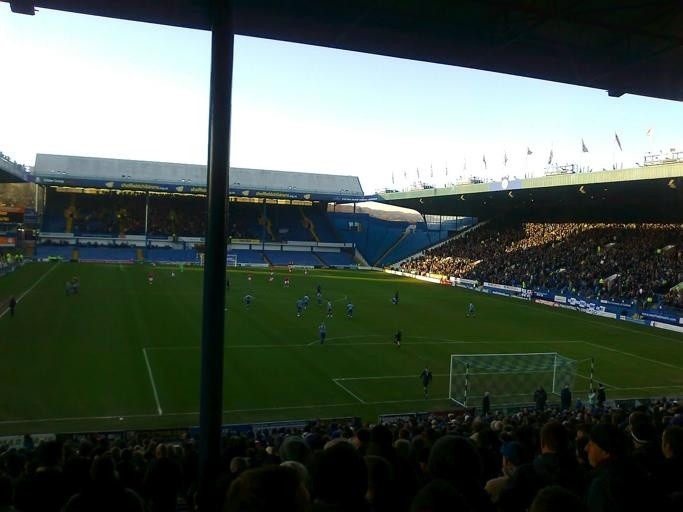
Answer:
420, 366, 432, 397
318, 322, 327, 344
389, 204, 683, 318
1, 383, 682, 512
0, 251, 24, 273
295, 283, 354, 320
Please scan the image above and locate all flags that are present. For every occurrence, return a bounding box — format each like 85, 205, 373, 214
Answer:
389, 127, 660, 181
